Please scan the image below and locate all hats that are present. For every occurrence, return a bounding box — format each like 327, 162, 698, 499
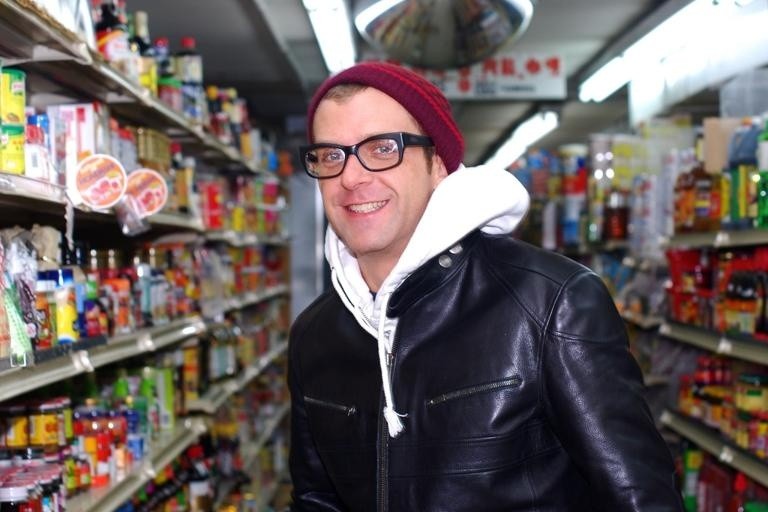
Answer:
306, 62, 464, 175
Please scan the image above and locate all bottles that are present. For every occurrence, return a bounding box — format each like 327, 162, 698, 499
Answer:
723, 251, 766, 339
98, 1, 242, 148
113, 367, 160, 442
31, 248, 168, 351
691, 356, 733, 422
604, 186, 627, 240
674, 117, 767, 229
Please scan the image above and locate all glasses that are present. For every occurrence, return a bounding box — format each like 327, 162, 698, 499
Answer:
298, 132, 434, 179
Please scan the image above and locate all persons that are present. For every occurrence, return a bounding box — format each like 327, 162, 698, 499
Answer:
287, 62, 688, 512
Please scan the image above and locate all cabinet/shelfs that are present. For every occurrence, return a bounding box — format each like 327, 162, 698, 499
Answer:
549, 230, 768, 511
1, 0, 292, 510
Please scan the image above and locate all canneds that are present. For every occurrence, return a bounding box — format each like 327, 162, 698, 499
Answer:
0, 69, 26, 123
0, 393, 148, 512
0, 124, 26, 175
